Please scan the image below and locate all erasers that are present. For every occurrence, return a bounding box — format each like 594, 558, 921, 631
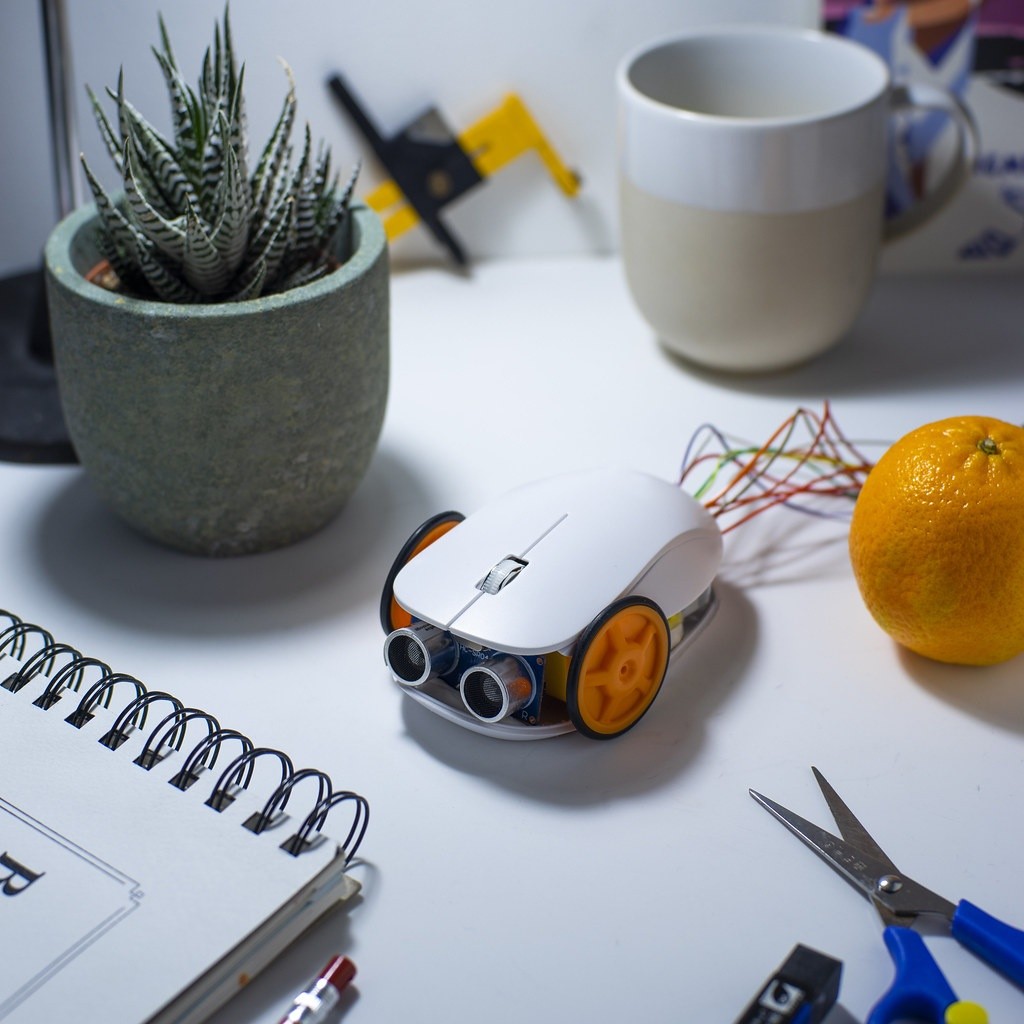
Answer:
735, 945, 842, 1024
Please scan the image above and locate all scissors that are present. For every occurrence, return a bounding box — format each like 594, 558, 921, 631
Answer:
749, 766, 1023, 1023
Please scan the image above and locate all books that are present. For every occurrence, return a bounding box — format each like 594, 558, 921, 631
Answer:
0, 608, 376, 1024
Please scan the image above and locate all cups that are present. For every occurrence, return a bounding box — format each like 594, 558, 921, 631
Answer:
616, 24, 894, 374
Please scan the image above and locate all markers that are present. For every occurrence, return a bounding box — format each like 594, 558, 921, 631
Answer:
278, 954, 356, 1023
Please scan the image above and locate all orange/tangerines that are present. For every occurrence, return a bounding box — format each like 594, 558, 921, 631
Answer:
848, 415, 1024, 665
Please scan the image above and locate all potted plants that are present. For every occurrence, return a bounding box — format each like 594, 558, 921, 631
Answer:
41, 1, 392, 556
